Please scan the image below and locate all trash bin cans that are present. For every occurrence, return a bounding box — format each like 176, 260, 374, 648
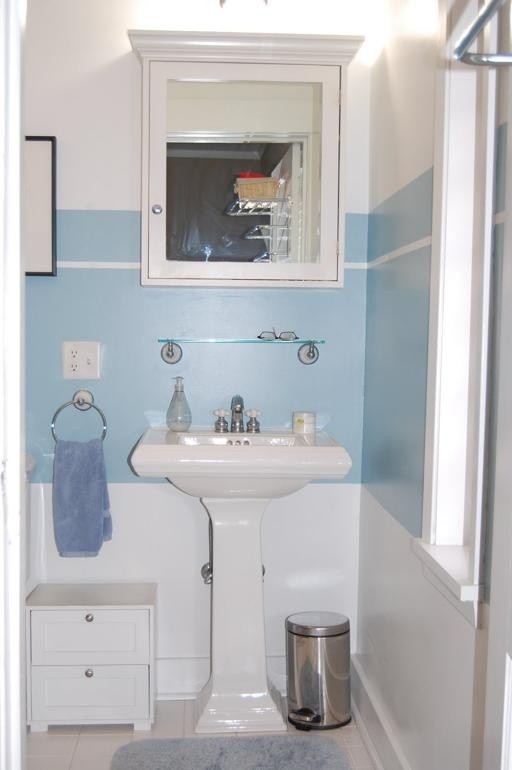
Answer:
284, 610, 352, 731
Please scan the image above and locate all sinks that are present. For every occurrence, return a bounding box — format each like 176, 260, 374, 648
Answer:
130, 427, 352, 499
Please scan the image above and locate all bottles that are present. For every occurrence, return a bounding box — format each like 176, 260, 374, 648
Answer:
291, 411, 316, 432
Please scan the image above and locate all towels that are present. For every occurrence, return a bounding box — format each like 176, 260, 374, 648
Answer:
53, 439, 113, 559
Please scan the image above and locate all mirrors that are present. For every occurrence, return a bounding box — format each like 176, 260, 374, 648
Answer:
164, 76, 324, 266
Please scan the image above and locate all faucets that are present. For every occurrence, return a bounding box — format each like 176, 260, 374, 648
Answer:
230, 395, 245, 432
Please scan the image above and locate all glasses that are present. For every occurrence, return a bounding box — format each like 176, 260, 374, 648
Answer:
258, 328, 299, 341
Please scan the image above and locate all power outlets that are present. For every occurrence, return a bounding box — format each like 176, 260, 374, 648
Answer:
64, 342, 100, 380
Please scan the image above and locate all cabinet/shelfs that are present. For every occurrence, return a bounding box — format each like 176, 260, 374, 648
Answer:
124, 26, 365, 288
24, 584, 159, 734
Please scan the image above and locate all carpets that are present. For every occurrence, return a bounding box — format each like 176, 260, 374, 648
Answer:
114, 735, 350, 769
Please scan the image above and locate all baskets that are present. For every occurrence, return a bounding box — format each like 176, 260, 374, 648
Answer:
236, 177, 279, 201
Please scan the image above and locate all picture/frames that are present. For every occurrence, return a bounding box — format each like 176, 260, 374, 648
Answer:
22, 134, 58, 277
